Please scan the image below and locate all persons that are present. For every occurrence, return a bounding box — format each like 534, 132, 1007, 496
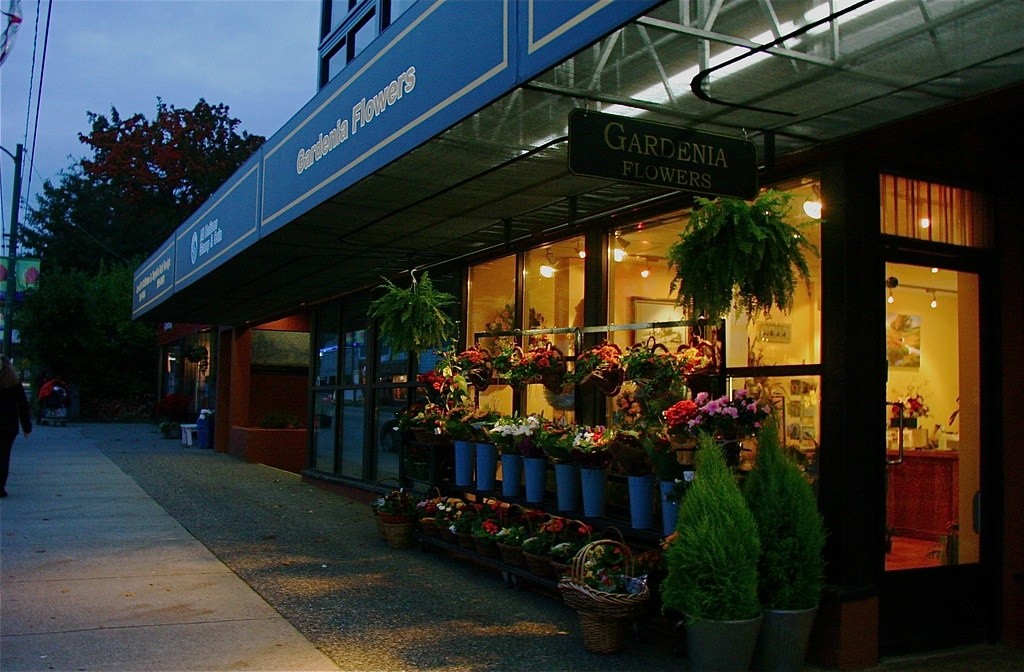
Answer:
0, 354, 33, 498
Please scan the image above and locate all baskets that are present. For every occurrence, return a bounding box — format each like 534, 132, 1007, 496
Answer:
469, 342, 706, 401
372, 485, 649, 653
669, 422, 720, 465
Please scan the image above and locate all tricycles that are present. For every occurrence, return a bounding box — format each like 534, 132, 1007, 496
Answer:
39, 380, 68, 427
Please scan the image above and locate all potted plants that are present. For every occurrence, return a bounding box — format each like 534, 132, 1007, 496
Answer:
744, 425, 832, 672
183, 340, 208, 376
159, 421, 180, 439
197, 412, 216, 449
414, 450, 448, 482
658, 426, 767, 672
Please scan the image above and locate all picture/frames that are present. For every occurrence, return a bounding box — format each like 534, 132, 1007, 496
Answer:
757, 320, 792, 344
629, 296, 693, 354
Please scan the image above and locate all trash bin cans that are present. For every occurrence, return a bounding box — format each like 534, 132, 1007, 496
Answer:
195, 409, 215, 449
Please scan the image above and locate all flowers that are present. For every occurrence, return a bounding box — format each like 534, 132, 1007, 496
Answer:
369, 342, 780, 592
892, 390, 930, 419
486, 301, 549, 348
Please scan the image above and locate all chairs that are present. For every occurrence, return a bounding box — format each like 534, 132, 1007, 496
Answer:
180, 409, 213, 446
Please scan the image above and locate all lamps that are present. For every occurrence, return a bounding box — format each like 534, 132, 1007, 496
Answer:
538, 260, 560, 279
574, 248, 586, 259
614, 242, 627, 263
804, 188, 821, 219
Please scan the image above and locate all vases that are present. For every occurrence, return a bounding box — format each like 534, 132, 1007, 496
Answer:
554, 463, 579, 512
474, 443, 498, 490
579, 467, 607, 518
403, 457, 416, 477
627, 475, 655, 529
424, 431, 451, 446
500, 452, 526, 497
660, 481, 680, 536
687, 373, 721, 399
378, 512, 412, 524
411, 426, 428, 442
453, 440, 476, 486
522, 458, 548, 503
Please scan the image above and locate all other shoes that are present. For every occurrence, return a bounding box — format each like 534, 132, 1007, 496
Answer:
0, 488, 7, 497
